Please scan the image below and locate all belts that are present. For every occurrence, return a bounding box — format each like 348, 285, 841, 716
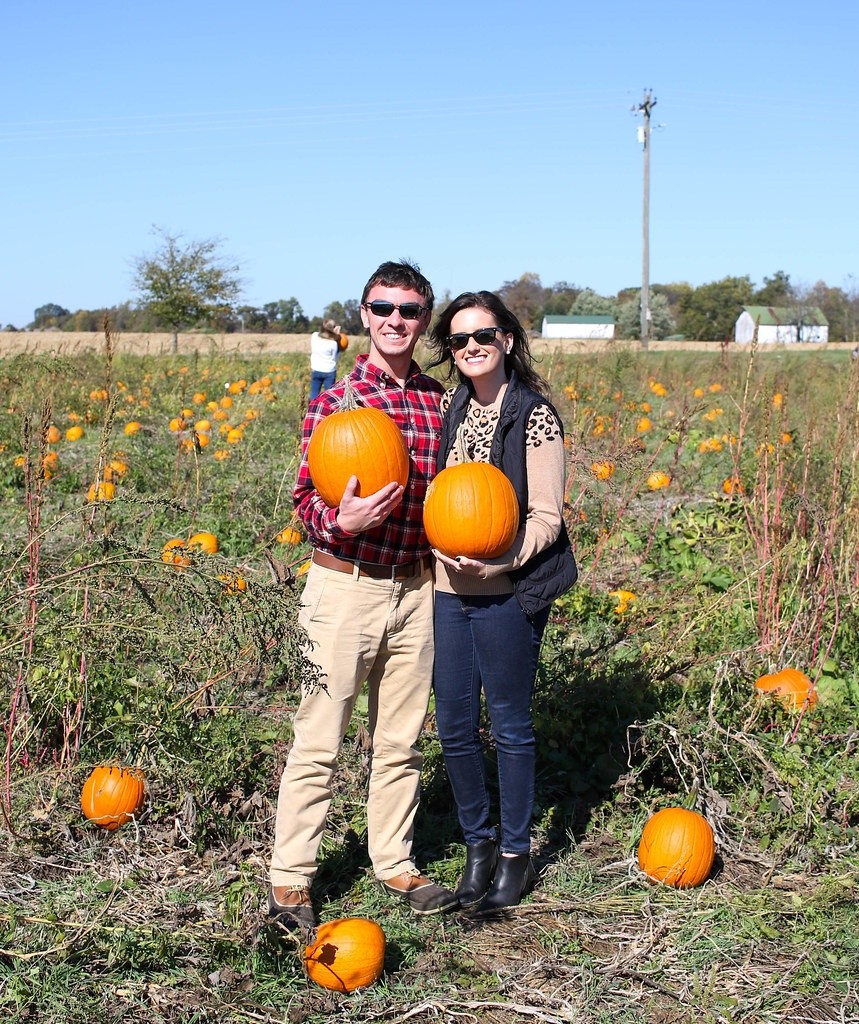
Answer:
312, 547, 433, 583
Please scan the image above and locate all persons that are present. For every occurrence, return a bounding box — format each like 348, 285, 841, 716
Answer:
431, 291, 577, 912
271, 261, 446, 930
310, 319, 341, 403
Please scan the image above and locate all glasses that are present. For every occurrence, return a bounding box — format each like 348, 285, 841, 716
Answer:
364, 299, 428, 319
446, 328, 506, 350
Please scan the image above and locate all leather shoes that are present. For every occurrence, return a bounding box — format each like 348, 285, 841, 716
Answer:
454, 839, 499, 908
475, 854, 530, 917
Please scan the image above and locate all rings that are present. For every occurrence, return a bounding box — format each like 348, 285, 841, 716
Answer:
448, 564, 451, 569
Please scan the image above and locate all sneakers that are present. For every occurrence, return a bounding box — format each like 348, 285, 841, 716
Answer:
383, 868, 458, 916
268, 884, 317, 928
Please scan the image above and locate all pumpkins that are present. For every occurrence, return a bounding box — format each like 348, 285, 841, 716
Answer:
549, 368, 797, 632
1, 335, 349, 688
309, 375, 410, 508
80, 751, 146, 830
422, 425, 519, 557
638, 779, 715, 888
300, 918, 386, 992
755, 662, 819, 714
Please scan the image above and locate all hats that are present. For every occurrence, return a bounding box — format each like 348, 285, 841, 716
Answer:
324, 319, 337, 333
